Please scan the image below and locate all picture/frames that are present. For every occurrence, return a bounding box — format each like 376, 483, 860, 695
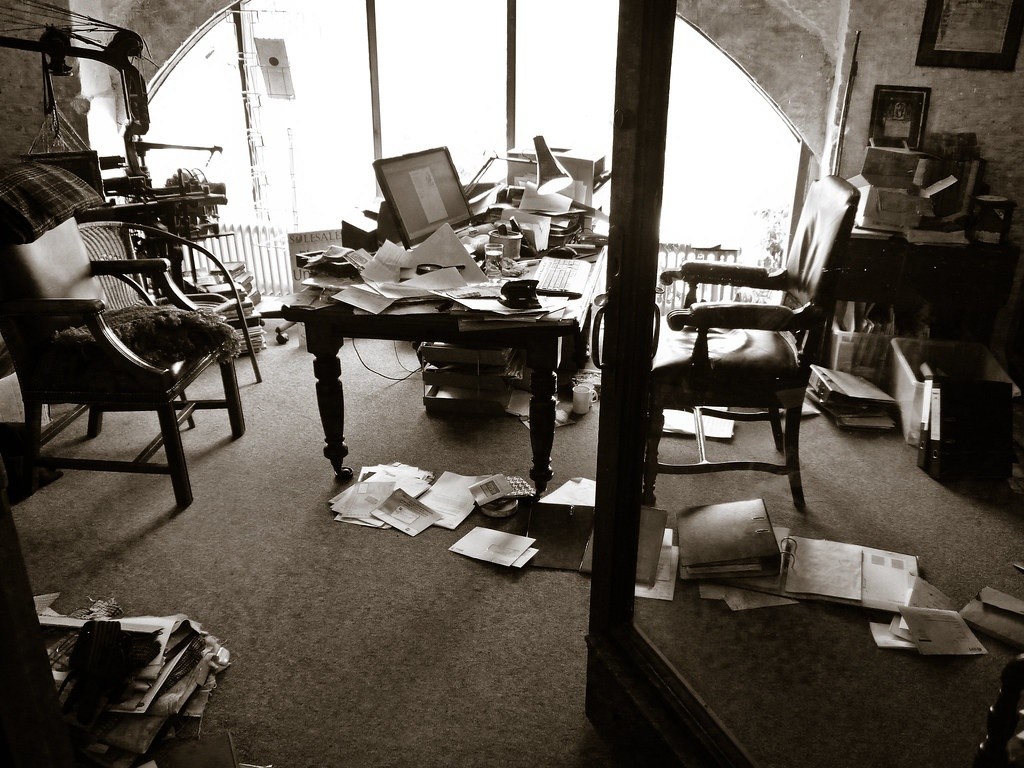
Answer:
867, 84, 933, 152
915, 0, 1024, 73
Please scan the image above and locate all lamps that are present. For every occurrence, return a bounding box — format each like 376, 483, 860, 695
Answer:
464, 135, 574, 198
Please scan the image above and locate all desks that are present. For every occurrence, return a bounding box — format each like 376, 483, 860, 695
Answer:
820, 231, 1022, 366
280, 224, 608, 501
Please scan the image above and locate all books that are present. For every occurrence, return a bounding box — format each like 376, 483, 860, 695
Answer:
182, 262, 268, 353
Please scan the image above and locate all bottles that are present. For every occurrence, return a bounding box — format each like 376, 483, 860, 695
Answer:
319, 287, 331, 304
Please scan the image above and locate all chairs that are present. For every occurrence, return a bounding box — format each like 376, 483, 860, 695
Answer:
640, 175, 862, 511
77, 222, 263, 429
0, 161, 245, 510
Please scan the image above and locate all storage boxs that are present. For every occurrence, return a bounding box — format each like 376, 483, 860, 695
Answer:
889, 338, 1022, 445
830, 303, 925, 388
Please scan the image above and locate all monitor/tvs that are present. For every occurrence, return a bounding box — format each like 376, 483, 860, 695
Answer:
373, 146, 475, 249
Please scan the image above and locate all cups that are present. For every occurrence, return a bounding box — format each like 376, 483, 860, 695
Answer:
573, 383, 598, 414
484, 243, 504, 278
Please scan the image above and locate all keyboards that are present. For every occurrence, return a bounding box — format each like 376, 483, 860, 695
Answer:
533, 254, 590, 297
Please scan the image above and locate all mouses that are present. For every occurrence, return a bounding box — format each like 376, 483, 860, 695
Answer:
547, 246, 577, 259
